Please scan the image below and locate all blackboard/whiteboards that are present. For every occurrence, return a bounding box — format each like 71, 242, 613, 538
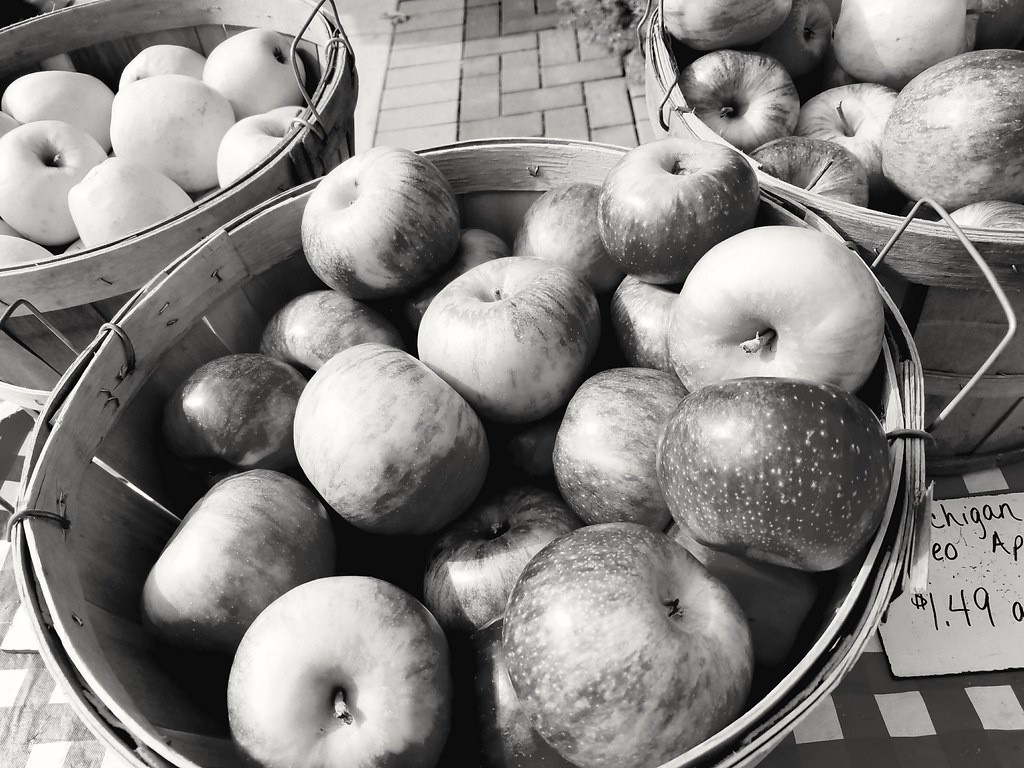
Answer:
878, 488, 1024, 678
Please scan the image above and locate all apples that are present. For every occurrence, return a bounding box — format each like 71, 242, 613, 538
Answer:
1, 27, 311, 270
137, 139, 891, 768
659, 0, 1024, 235
